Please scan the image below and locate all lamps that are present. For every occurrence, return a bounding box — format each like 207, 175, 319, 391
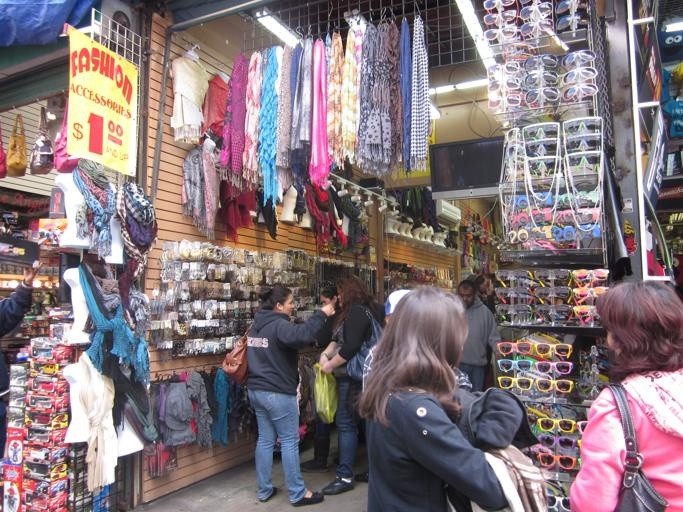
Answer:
344, 9, 442, 120
328, 172, 401, 216
251, 6, 304, 48
466, 218, 506, 249
662, 18, 683, 33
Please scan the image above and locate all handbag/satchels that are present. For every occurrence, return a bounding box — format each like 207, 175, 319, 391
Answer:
610, 385, 669, 512
222, 335, 248, 384
346, 317, 384, 381
313, 363, 338, 424
0, 97, 80, 177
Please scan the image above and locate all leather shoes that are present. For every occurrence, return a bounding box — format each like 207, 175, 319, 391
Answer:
259, 461, 369, 507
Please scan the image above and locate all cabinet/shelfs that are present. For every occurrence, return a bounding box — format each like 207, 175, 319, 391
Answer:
490, 29, 622, 483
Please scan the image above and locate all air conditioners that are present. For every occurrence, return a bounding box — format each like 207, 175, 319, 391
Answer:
436, 199, 461, 226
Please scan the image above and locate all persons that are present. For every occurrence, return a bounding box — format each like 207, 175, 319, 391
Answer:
302, 283, 348, 473
61, 351, 114, 445
182, 137, 226, 218
387, 210, 449, 249
347, 285, 474, 413
223, 284, 338, 507
320, 273, 382, 495
570, 280, 682, 512
357, 285, 524, 512
62, 255, 111, 346
54, 171, 114, 249
106, 183, 144, 266
283, 179, 356, 240
1, 257, 44, 460
112, 366, 149, 458
447, 278, 500, 392
171, 50, 207, 146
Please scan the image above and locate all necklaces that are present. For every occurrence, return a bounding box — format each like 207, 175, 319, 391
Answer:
492, 117, 603, 235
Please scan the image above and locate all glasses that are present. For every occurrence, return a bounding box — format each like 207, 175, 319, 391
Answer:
497, 342, 574, 394
523, 413, 588, 511
499, 116, 604, 243
482, 0, 598, 108
494, 268, 610, 325
